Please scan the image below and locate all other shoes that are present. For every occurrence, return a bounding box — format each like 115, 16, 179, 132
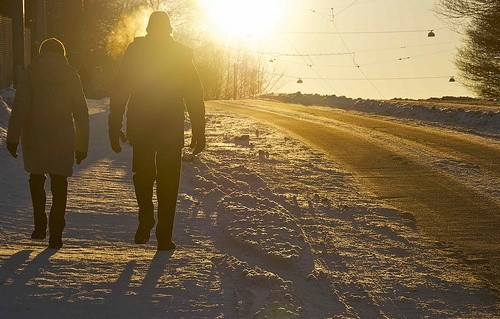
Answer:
157, 240, 176, 251
31, 230, 47, 239
49, 239, 63, 249
135, 218, 155, 244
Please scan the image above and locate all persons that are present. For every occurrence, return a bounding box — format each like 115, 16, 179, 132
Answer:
6, 38, 89, 249
108, 10, 205, 251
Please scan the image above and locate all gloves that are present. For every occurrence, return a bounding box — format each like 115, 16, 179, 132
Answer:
109, 126, 125, 153
7, 142, 18, 158
75, 151, 87, 164
190, 132, 205, 155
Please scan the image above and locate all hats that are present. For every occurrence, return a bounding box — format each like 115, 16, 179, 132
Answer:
146, 11, 172, 34
38, 38, 65, 56
